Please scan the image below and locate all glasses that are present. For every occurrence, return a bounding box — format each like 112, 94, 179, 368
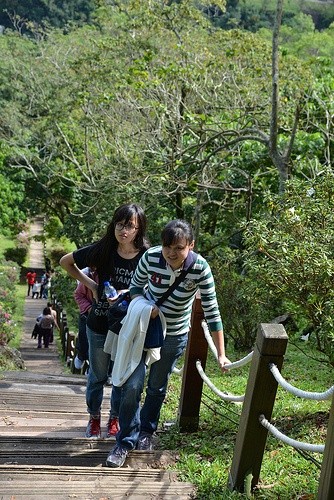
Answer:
114, 222, 139, 232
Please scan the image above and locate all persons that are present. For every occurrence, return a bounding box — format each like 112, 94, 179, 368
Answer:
36, 307, 60, 348
59, 203, 151, 437
74, 241, 114, 386
106, 219, 232, 468
26, 269, 54, 299
42, 302, 57, 345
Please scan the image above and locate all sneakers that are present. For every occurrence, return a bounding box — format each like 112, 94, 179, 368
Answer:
106, 444, 136, 468
85, 414, 101, 439
136, 435, 152, 451
74, 353, 85, 369
105, 416, 121, 437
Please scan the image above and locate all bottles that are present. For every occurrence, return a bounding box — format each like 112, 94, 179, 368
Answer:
104, 281, 120, 307
118, 300, 128, 312
